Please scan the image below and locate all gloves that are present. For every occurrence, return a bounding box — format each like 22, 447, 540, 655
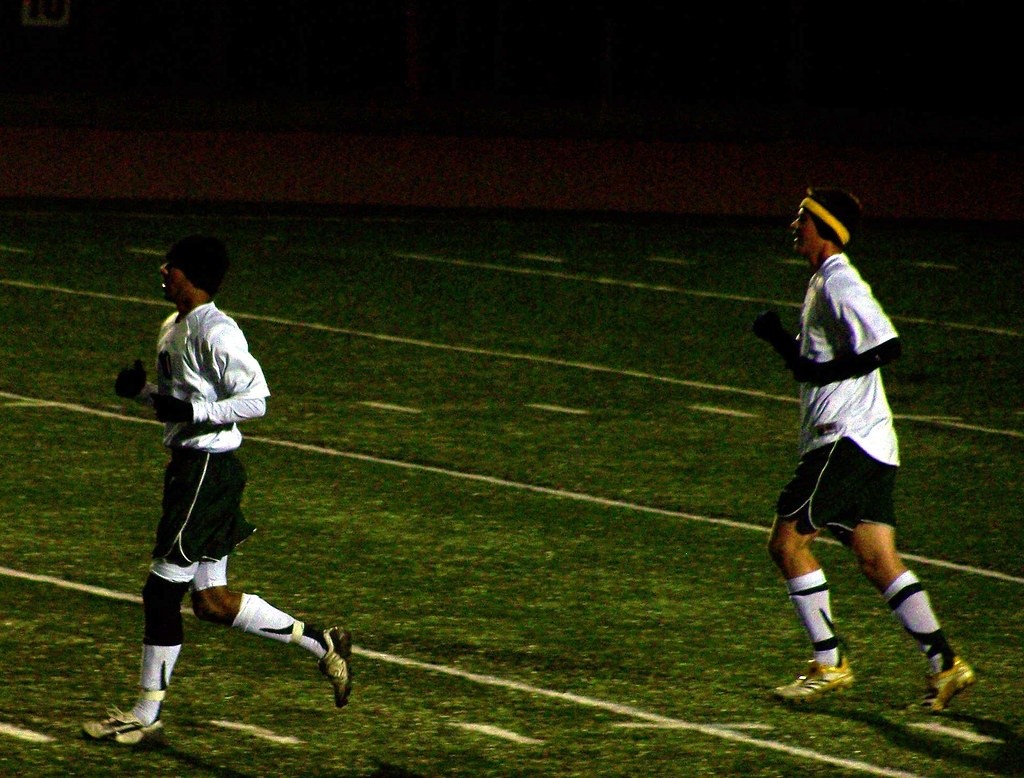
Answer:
149, 392, 193, 424
752, 311, 801, 372
792, 337, 902, 388
114, 360, 147, 400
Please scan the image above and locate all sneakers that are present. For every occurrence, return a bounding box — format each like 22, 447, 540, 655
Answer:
905, 655, 977, 715
81, 703, 165, 746
772, 650, 857, 704
319, 626, 354, 709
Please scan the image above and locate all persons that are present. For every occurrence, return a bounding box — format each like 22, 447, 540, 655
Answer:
753, 189, 977, 714
83, 236, 353, 745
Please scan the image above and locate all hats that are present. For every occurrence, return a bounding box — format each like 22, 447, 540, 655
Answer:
165, 234, 231, 298
